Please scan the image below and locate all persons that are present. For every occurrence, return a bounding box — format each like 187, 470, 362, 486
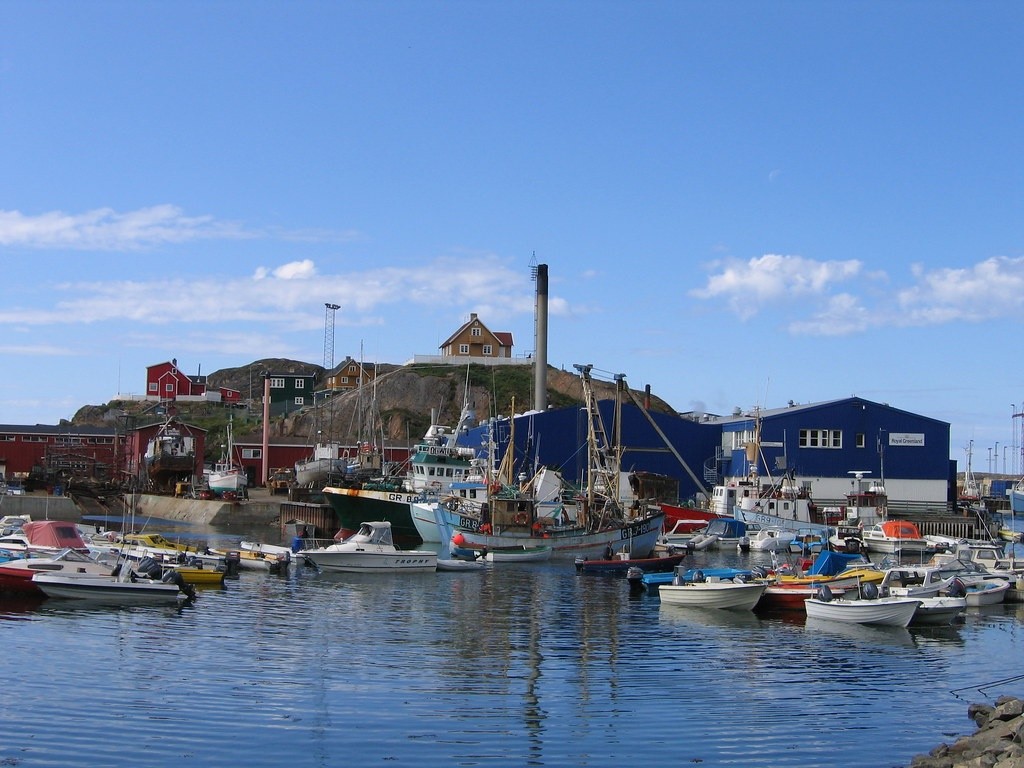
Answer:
604, 540, 613, 561
362, 525, 373, 542
292, 531, 305, 552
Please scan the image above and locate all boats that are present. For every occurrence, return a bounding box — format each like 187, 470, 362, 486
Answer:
204, 541, 302, 571
641, 565, 752, 584
658, 583, 769, 610
805, 617, 915, 646
659, 606, 759, 627
221, 490, 237, 500
437, 547, 553, 569
203, 415, 247, 498
574, 554, 687, 579
297, 517, 437, 573
200, 489, 215, 499
655, 406, 1024, 624
0, 488, 240, 606
803, 554, 925, 628
295, 304, 666, 559
1011, 476, 1024, 513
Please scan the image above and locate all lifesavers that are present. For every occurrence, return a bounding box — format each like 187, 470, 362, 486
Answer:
604, 547, 613, 560
514, 511, 528, 525
876, 505, 886, 517
